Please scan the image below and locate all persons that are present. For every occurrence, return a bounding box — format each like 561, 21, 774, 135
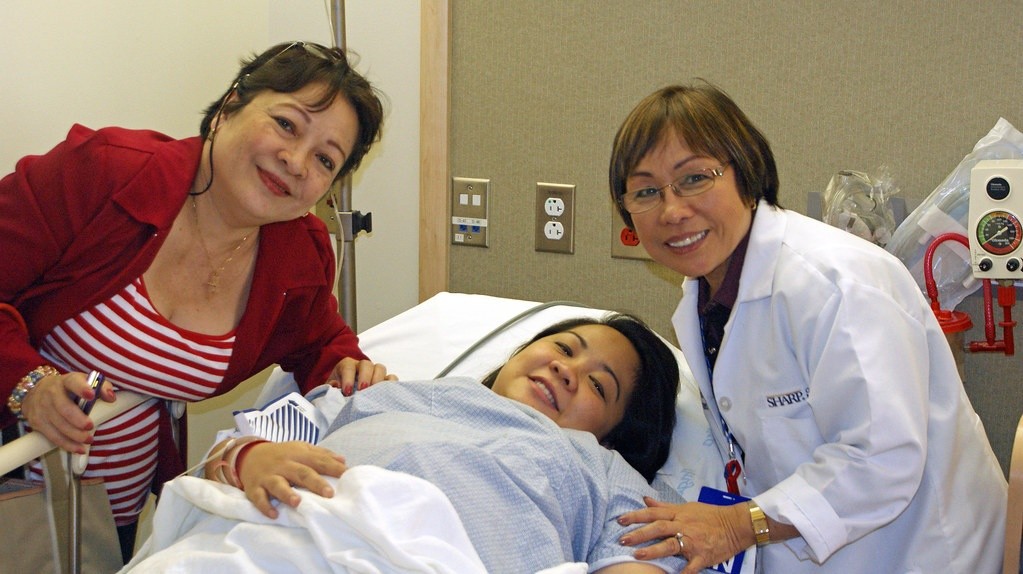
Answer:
608, 76, 1009, 574
204, 310, 690, 574
0, 42, 398, 566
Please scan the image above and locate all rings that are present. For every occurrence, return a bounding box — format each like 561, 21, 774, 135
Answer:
674, 533, 684, 556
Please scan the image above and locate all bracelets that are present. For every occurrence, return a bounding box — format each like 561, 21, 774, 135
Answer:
212, 436, 271, 491
748, 500, 770, 548
7, 366, 61, 422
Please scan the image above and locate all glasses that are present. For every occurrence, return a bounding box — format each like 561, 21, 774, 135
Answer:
618, 161, 730, 213
244, 39, 336, 76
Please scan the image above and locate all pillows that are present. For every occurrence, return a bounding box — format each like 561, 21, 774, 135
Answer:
254, 292, 729, 499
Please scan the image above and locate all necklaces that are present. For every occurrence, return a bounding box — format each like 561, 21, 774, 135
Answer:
191, 186, 259, 300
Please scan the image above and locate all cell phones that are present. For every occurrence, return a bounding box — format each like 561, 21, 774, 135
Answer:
77, 370, 104, 416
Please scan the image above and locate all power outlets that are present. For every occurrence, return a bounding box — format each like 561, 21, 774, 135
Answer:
535, 182, 576, 254
450, 176, 491, 248
610, 200, 653, 261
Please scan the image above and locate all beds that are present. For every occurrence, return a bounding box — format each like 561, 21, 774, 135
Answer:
0, 391, 757, 574
